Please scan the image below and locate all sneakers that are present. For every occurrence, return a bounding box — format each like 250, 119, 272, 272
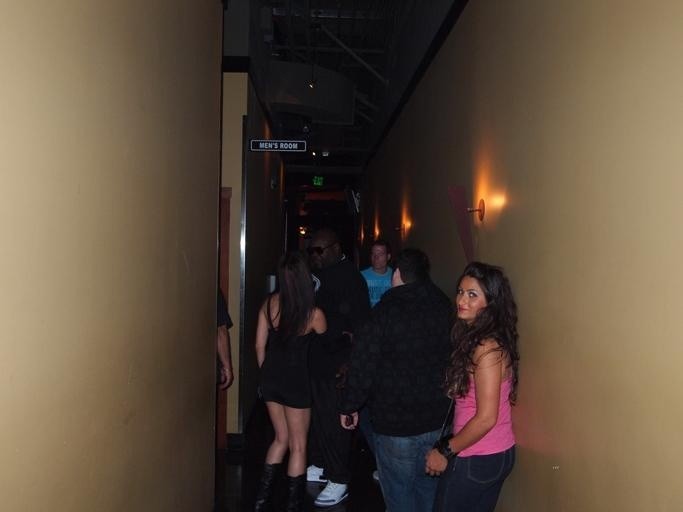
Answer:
314, 480, 348, 506
306, 465, 328, 483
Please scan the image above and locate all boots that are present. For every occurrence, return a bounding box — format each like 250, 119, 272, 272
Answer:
255, 462, 281, 510
286, 474, 305, 511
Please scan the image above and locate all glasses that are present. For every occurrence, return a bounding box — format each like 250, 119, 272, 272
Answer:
310, 246, 333, 256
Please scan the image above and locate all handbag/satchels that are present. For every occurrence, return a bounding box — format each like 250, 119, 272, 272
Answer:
427, 434, 453, 477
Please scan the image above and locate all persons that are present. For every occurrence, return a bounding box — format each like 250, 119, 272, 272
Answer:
251, 227, 519, 511
217, 286, 234, 396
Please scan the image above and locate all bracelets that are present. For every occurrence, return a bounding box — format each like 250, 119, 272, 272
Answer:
221, 368, 233, 372
